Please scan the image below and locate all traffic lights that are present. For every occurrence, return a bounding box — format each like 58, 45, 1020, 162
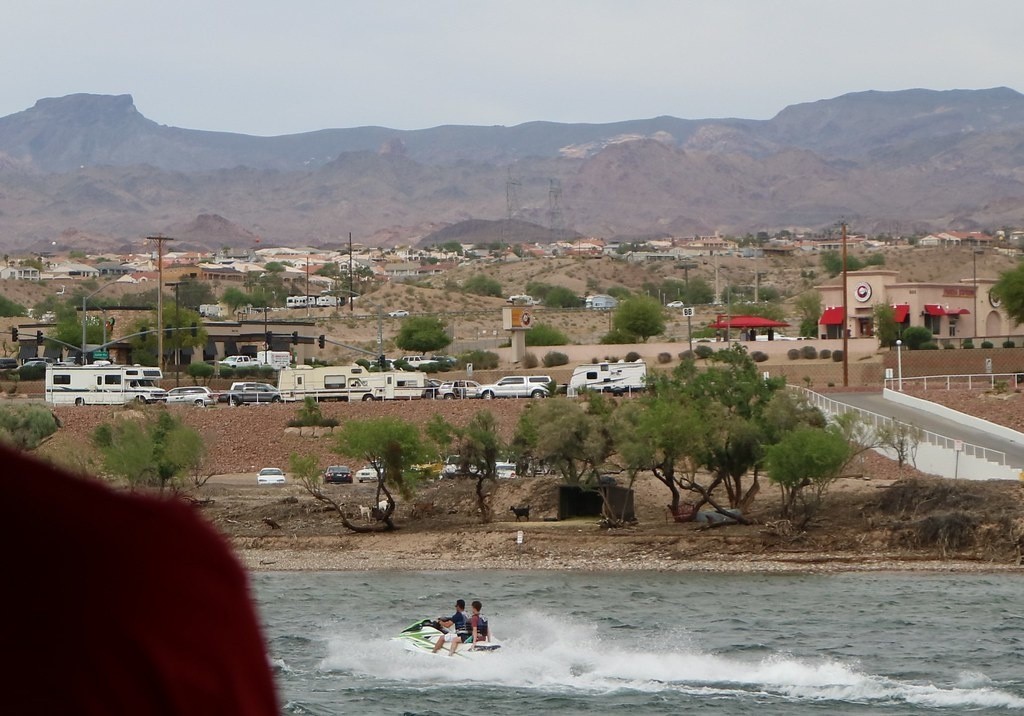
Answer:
319, 335, 324, 349
265, 330, 272, 344
37, 330, 43, 345
140, 327, 146, 341
165, 324, 171, 339
190, 322, 198, 336
109, 318, 115, 325
12, 327, 18, 341
380, 354, 385, 368
292, 332, 299, 345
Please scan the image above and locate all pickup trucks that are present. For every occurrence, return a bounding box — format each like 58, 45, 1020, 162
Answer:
166, 382, 279, 405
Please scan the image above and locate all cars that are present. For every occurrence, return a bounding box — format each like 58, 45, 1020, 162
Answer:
666, 300, 684, 308
0, 357, 80, 371
219, 350, 293, 369
318, 454, 548, 485
390, 309, 410, 317
256, 468, 287, 486
369, 356, 459, 368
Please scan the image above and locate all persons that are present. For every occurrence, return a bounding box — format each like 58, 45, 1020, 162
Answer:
716, 330, 721, 342
745, 327, 756, 341
448, 601, 491, 656
432, 600, 473, 653
768, 327, 774, 341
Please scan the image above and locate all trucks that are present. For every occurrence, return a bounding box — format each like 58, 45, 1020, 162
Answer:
585, 294, 618, 310
45, 361, 168, 404
278, 363, 439, 401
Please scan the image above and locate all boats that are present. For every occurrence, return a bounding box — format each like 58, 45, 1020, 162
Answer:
401, 619, 501, 651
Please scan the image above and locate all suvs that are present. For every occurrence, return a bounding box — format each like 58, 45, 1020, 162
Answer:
433, 375, 558, 399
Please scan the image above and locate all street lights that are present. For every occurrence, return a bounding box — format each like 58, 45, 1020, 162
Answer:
674, 262, 697, 353
256, 307, 272, 365
974, 249, 985, 336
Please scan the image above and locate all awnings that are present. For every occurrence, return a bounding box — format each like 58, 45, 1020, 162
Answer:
819, 307, 845, 325
925, 305, 971, 315
888, 305, 909, 322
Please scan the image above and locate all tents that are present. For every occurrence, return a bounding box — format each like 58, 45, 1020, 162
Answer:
708, 316, 790, 329
